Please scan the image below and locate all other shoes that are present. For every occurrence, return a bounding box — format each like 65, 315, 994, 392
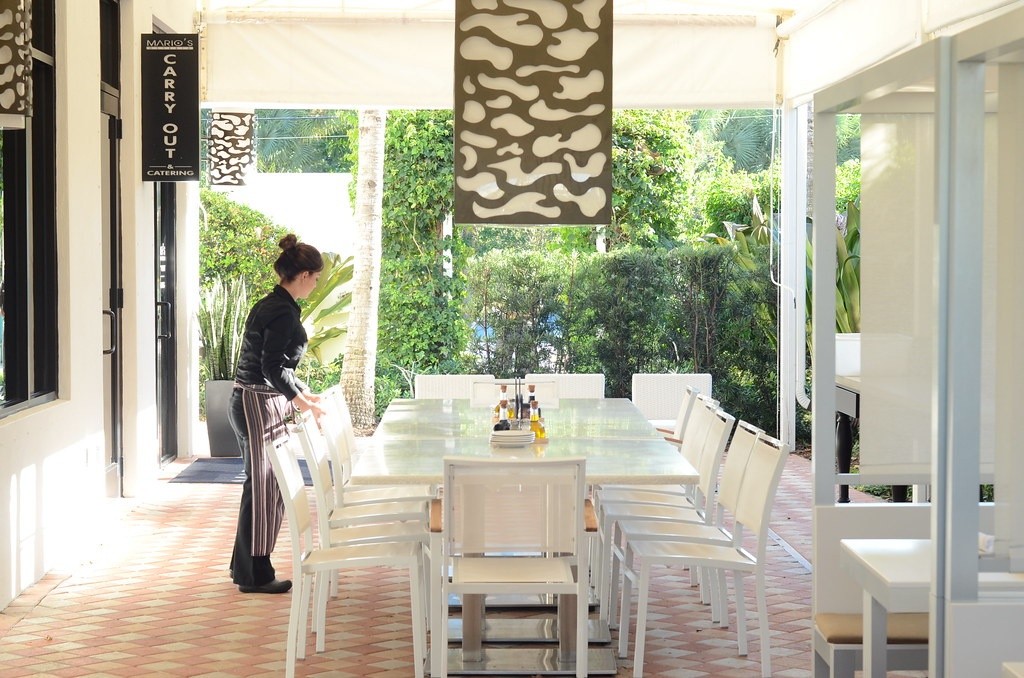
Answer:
238, 578, 292, 593
229, 570, 233, 578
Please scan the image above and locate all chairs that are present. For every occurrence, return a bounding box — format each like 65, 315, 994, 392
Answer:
264, 368, 791, 678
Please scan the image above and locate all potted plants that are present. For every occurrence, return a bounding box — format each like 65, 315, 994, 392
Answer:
702, 191, 861, 378
198, 271, 251, 456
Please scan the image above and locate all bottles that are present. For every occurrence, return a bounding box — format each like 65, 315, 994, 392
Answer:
491, 381, 546, 441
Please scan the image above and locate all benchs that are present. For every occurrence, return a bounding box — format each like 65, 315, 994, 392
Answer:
812, 501, 993, 678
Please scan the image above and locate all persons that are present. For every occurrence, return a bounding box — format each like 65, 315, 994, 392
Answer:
225, 233, 325, 595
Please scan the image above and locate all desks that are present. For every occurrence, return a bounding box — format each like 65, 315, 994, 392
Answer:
836, 374, 930, 504
838, 536, 994, 678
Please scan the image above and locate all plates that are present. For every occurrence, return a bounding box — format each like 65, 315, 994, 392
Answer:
487, 427, 535, 449
